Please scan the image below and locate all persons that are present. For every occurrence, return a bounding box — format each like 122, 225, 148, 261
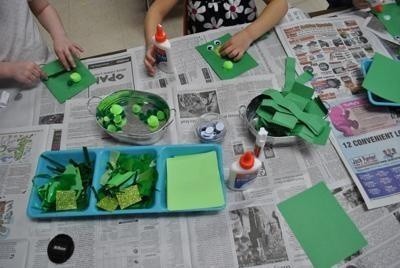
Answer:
0, 0, 83, 89
143, 1, 288, 76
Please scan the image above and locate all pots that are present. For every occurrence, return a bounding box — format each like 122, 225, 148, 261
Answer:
86, 90, 176, 146
240, 93, 332, 148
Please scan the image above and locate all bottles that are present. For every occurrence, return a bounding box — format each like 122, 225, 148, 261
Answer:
254, 126, 268, 158
228, 152, 263, 189
151, 24, 176, 74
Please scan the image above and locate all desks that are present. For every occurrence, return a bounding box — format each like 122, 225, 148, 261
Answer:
0, 9, 400, 268
